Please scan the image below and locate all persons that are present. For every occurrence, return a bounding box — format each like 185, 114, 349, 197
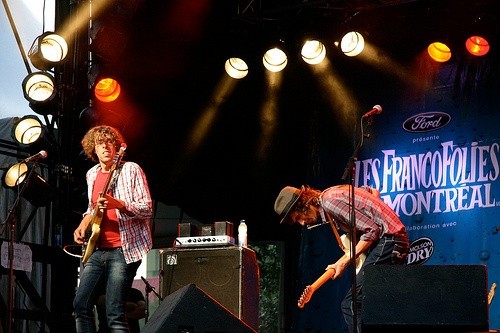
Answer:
97, 275, 145, 333
273, 185, 410, 333
73, 126, 153, 333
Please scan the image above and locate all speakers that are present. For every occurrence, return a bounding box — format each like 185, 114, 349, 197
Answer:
157, 247, 259, 329
360, 264, 490, 333
139, 282, 259, 332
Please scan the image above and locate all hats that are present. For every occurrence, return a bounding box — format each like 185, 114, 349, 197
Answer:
274, 185, 306, 224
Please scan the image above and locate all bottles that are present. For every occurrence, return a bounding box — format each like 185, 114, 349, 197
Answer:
238, 220, 249, 247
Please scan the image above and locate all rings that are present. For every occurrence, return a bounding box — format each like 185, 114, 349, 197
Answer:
102, 203, 104, 206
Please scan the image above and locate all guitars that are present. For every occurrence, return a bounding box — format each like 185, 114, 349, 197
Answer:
81, 143, 127, 267
297, 233, 370, 309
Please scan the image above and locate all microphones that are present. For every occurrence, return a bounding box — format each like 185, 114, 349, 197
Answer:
21, 150, 47, 164
362, 104, 383, 119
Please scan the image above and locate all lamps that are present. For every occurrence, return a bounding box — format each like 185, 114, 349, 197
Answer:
1, 161, 55, 208
224, 42, 251, 80
334, 19, 365, 58
88, 60, 122, 103
28, 31, 70, 71
87, 22, 131, 62
261, 35, 288, 74
22, 73, 61, 116
297, 28, 327, 65
10, 115, 60, 162
428, 32, 455, 63
464, 19, 491, 56
